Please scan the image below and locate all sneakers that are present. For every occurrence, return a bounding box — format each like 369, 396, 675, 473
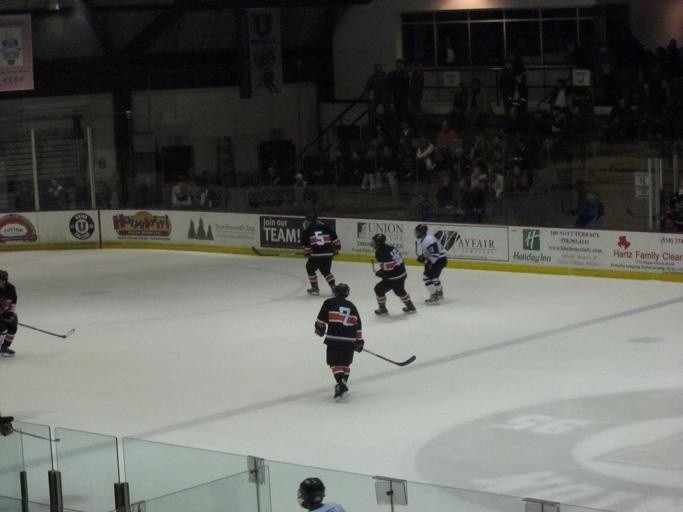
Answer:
306, 284, 318, 293
437, 289, 442, 297
402, 301, 415, 312
332, 382, 348, 397
0, 343, 15, 354
425, 293, 438, 302
374, 304, 388, 314
331, 283, 335, 291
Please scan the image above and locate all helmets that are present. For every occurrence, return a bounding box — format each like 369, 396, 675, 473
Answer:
1, 268, 10, 287
414, 224, 428, 239
335, 283, 350, 296
305, 213, 319, 225
370, 234, 386, 250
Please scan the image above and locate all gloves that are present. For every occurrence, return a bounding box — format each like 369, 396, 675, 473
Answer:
355, 342, 365, 351
418, 256, 425, 263
376, 271, 383, 279
316, 330, 324, 337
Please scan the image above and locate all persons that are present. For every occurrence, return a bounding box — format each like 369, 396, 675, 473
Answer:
467, 167, 486, 187
660, 191, 683, 234
292, 170, 312, 198
434, 119, 454, 146
417, 137, 446, 171
486, 162, 505, 202
258, 163, 281, 191
501, 62, 528, 133
0, 269, 18, 357
370, 233, 417, 315
448, 82, 469, 121
466, 133, 504, 172
548, 105, 572, 162
537, 79, 589, 108
453, 188, 493, 224
357, 62, 389, 137
413, 223, 449, 304
572, 193, 603, 229
443, 36, 458, 67
16, 181, 37, 210
374, 145, 403, 196
353, 150, 376, 191
509, 162, 534, 194
589, 39, 683, 144
330, 146, 353, 179
408, 57, 425, 119
43, 179, 69, 211
389, 59, 409, 109
296, 477, 345, 512
315, 283, 365, 402
299, 212, 342, 296
433, 174, 457, 205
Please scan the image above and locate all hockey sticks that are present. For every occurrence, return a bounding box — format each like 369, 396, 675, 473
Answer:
414, 241, 420, 258
18, 322, 76, 338
370, 259, 377, 273
252, 247, 309, 257
362, 348, 418, 366
13, 429, 62, 442
